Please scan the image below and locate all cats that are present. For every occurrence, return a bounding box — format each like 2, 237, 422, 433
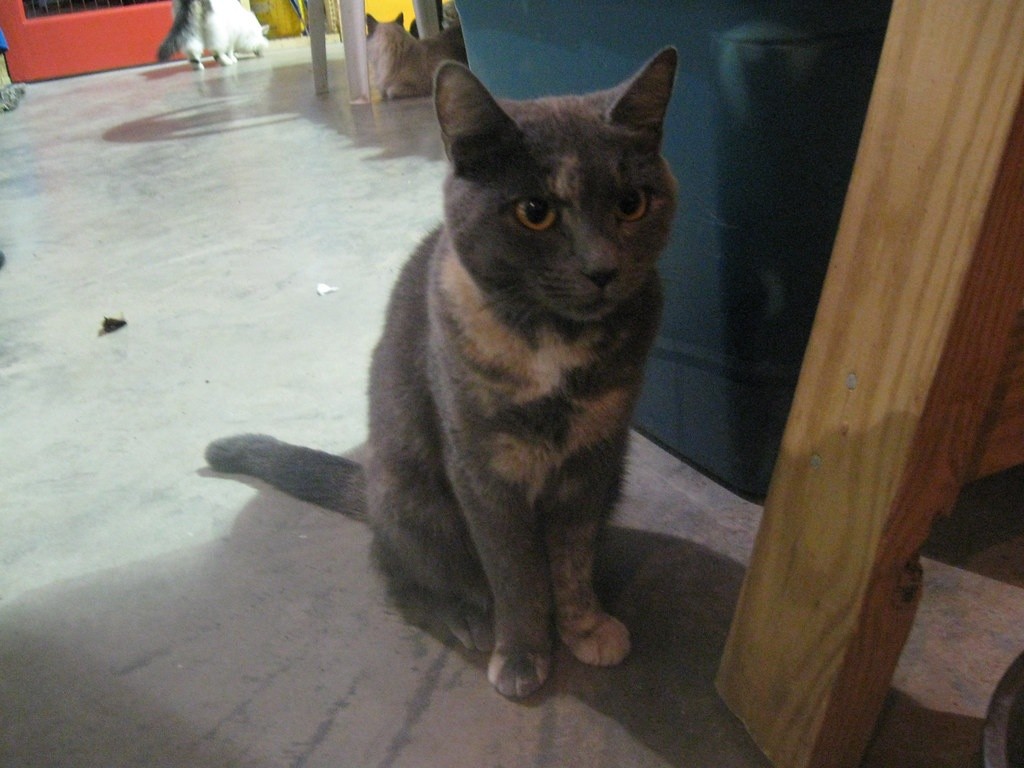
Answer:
156, 0, 271, 71
204, 41, 681, 700
365, 12, 469, 101
